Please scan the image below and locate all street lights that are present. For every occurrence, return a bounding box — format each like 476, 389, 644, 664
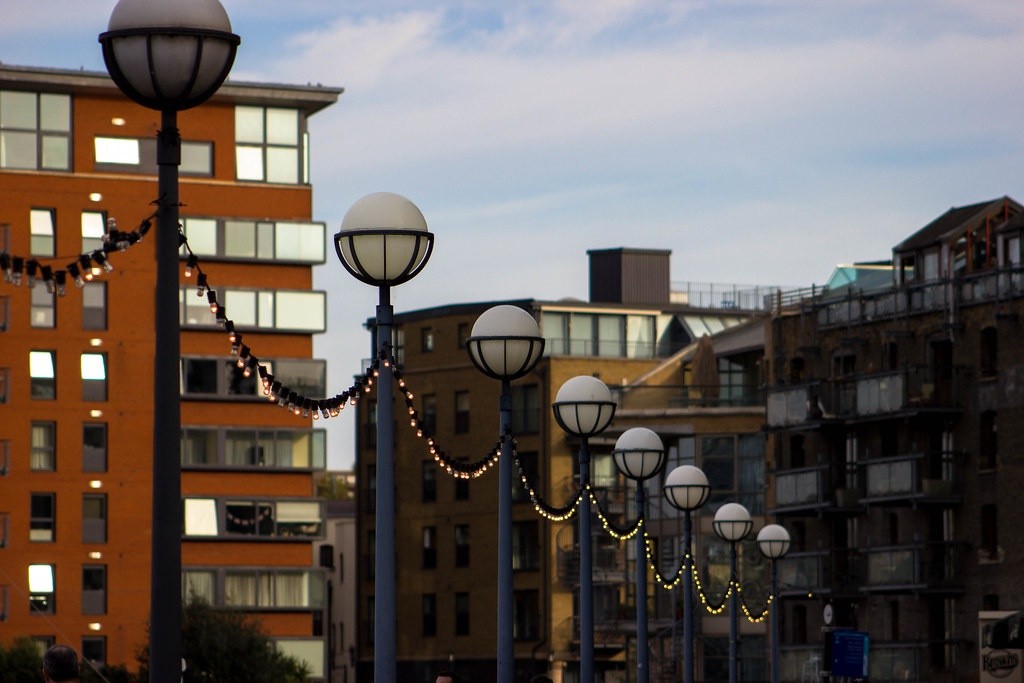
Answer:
663, 465, 708, 683
464, 304, 547, 683
711, 503, 754, 683
551, 375, 619, 682
99, 0, 242, 681
756, 523, 792, 683
333, 193, 434, 682
609, 427, 666, 683
